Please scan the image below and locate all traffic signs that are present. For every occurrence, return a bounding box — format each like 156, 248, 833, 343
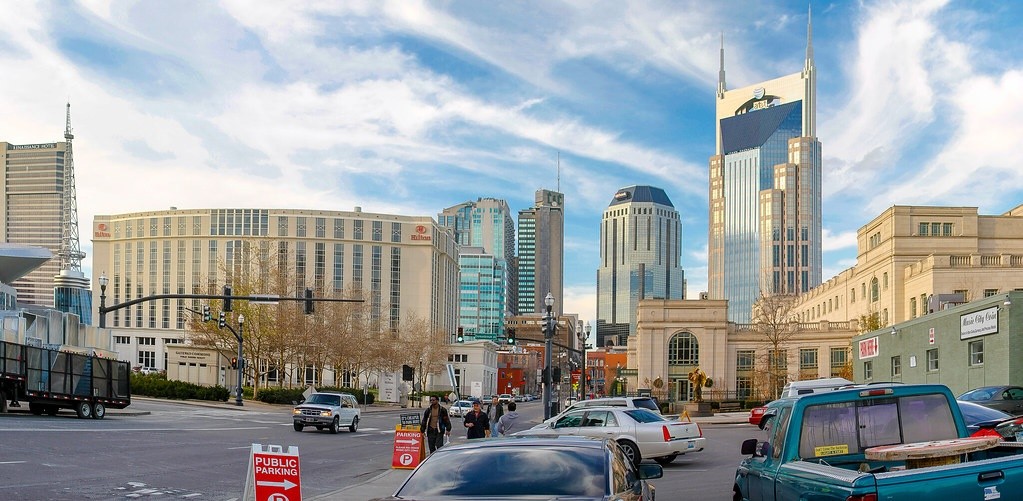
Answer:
392, 430, 422, 469
252, 451, 302, 501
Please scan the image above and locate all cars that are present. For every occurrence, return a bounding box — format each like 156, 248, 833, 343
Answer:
510, 405, 706, 469
956, 385, 1023, 416
449, 400, 475, 418
468, 393, 541, 405
367, 436, 664, 501
749, 400, 773, 427
953, 399, 1023, 442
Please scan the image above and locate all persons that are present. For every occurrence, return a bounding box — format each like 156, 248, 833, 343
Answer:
498, 402, 519, 437
444, 393, 449, 404
487, 396, 504, 437
464, 400, 490, 439
421, 395, 452, 454
690, 367, 703, 401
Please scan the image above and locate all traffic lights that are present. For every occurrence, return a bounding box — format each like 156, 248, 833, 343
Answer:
587, 375, 591, 384
219, 312, 226, 329
231, 358, 237, 368
203, 305, 211, 324
458, 327, 464, 342
541, 315, 548, 333
507, 329, 515, 344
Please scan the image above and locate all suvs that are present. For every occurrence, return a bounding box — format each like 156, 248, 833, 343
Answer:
293, 391, 361, 434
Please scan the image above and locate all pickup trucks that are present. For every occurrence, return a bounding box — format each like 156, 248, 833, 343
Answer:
732, 384, 1023, 501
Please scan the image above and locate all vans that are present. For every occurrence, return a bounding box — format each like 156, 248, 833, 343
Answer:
543, 395, 663, 424
780, 377, 856, 399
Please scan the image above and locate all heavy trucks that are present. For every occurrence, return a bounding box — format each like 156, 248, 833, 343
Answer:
0, 340, 132, 420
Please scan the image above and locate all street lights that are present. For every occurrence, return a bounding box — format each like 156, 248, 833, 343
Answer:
419, 357, 422, 408
575, 320, 592, 401
235, 313, 245, 406
97, 270, 109, 328
462, 367, 466, 400
490, 371, 493, 397
543, 288, 555, 422
